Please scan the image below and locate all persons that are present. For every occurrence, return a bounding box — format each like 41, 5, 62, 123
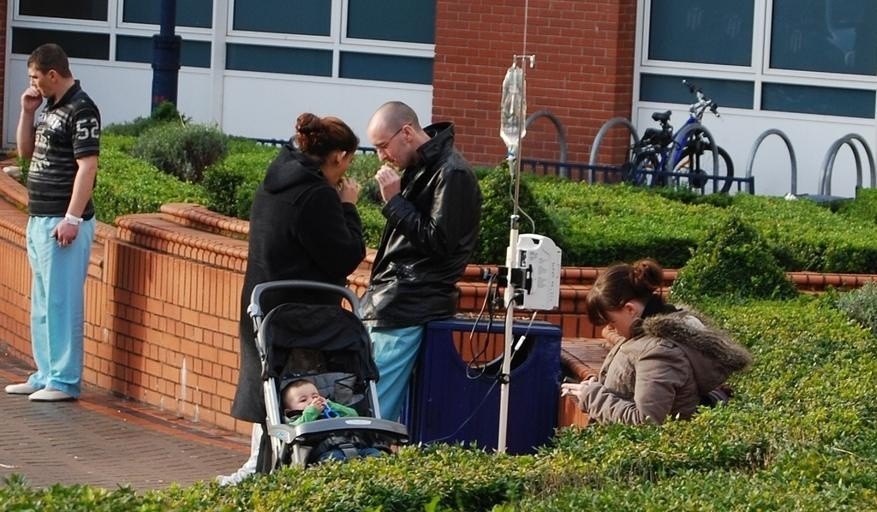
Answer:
5, 43, 101, 401
560, 260, 753, 427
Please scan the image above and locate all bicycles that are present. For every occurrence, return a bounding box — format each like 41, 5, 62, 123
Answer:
629, 78, 736, 199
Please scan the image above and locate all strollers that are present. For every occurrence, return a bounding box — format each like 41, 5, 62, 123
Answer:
246, 278, 413, 476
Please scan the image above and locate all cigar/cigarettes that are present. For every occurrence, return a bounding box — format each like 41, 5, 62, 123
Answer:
560, 388, 570, 397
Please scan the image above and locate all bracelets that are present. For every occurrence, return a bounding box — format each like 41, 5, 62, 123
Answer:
65, 213, 83, 225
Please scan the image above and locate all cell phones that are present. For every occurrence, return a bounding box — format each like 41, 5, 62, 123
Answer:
563, 375, 580, 383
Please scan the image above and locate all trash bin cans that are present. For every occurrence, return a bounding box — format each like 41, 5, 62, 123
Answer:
401, 318, 560, 455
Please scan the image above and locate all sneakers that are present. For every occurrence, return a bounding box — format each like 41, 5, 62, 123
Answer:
5, 381, 73, 401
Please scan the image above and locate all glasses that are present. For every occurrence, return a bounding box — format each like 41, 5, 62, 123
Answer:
373, 123, 411, 150
